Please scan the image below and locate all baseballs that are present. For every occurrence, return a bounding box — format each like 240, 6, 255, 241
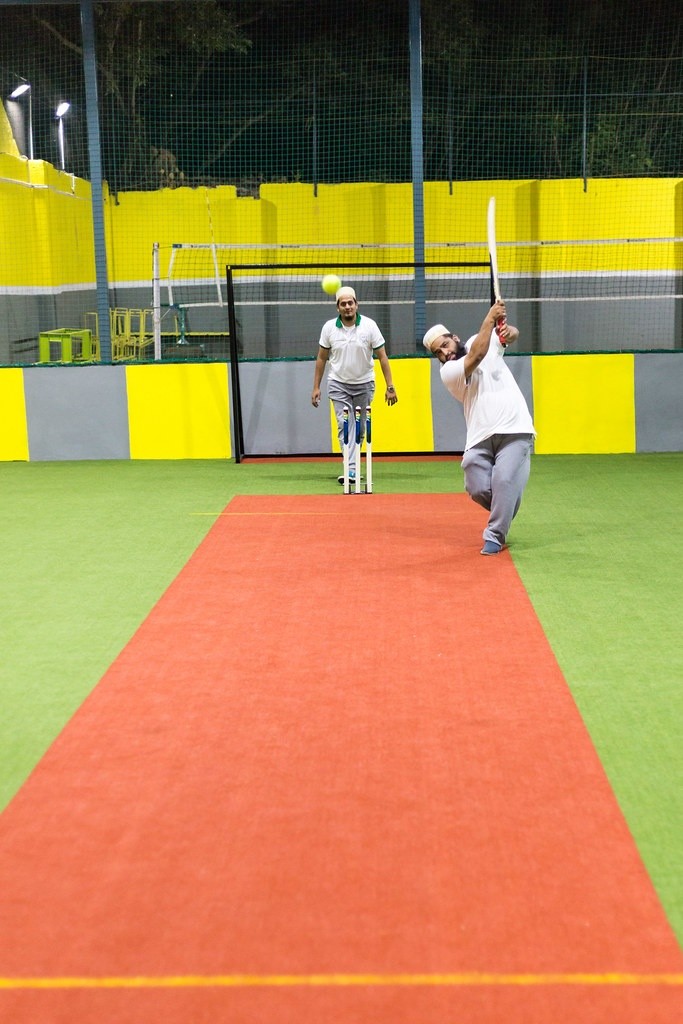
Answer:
320, 273, 342, 295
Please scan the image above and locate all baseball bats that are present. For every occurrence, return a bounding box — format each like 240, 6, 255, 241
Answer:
485, 197, 508, 344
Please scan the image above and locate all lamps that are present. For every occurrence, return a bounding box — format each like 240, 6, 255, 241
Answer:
5, 71, 30, 99
51, 95, 70, 119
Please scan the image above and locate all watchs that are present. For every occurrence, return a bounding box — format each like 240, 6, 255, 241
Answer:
387, 385, 395, 392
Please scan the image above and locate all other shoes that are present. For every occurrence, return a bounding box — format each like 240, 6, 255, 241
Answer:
481, 539, 501, 555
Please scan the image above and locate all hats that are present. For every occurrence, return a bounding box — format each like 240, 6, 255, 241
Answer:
336, 287, 356, 304
423, 324, 451, 352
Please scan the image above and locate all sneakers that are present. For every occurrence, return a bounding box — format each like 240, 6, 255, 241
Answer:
338, 471, 355, 483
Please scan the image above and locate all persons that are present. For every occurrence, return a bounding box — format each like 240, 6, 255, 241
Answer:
421, 299, 537, 557
312, 285, 398, 486
